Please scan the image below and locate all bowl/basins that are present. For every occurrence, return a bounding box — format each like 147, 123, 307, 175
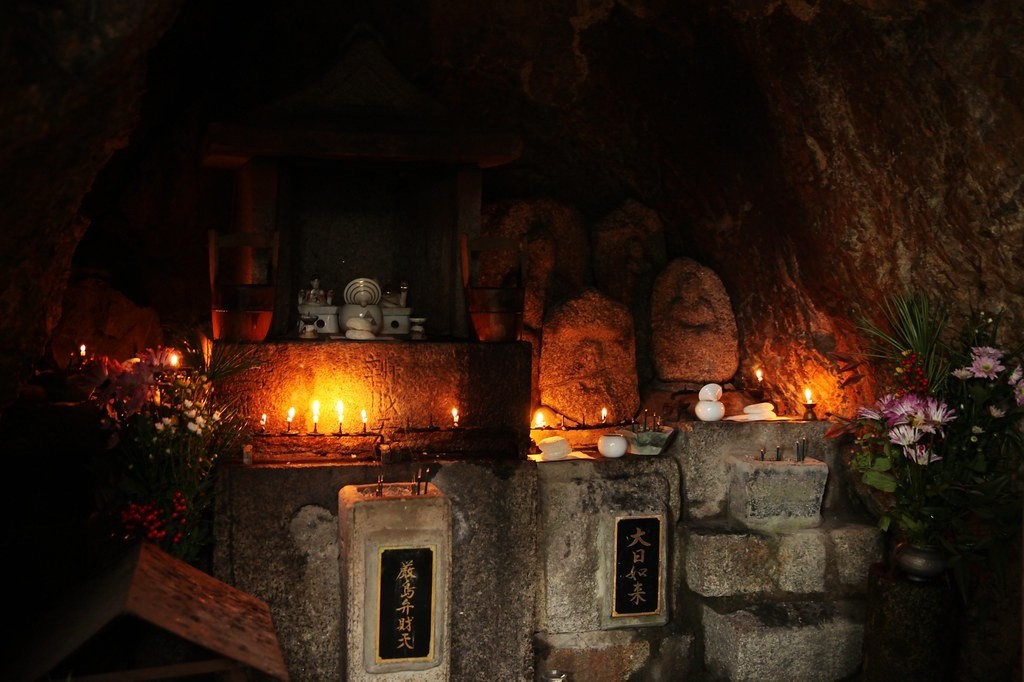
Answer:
696, 400, 725, 422
598, 434, 628, 458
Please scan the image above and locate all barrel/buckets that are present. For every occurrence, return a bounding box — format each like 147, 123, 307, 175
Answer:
211, 282, 276, 344
463, 282, 524, 342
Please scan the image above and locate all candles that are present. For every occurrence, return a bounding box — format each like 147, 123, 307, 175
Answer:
362, 417, 367, 424
757, 377, 763, 382
260, 420, 265, 425
313, 416, 318, 423
287, 418, 292, 422
80, 351, 86, 356
338, 415, 343, 423
453, 416, 460, 427
536, 422, 544, 428
807, 400, 812, 404
